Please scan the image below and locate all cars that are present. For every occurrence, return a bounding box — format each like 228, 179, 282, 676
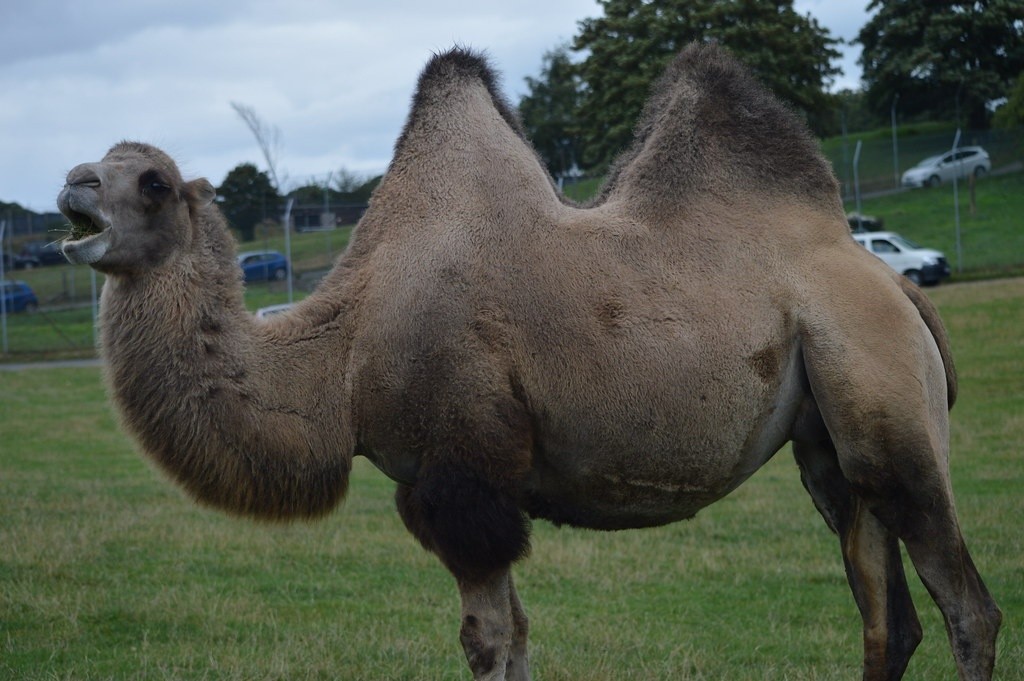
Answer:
848, 214, 882, 233
556, 161, 584, 179
0, 241, 69, 271
0, 280, 37, 316
236, 250, 289, 283
851, 230, 947, 288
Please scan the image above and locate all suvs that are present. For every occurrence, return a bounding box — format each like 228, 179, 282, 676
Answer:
901, 147, 990, 190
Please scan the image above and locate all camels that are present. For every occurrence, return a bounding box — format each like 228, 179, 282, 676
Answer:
58, 40, 1003, 681
